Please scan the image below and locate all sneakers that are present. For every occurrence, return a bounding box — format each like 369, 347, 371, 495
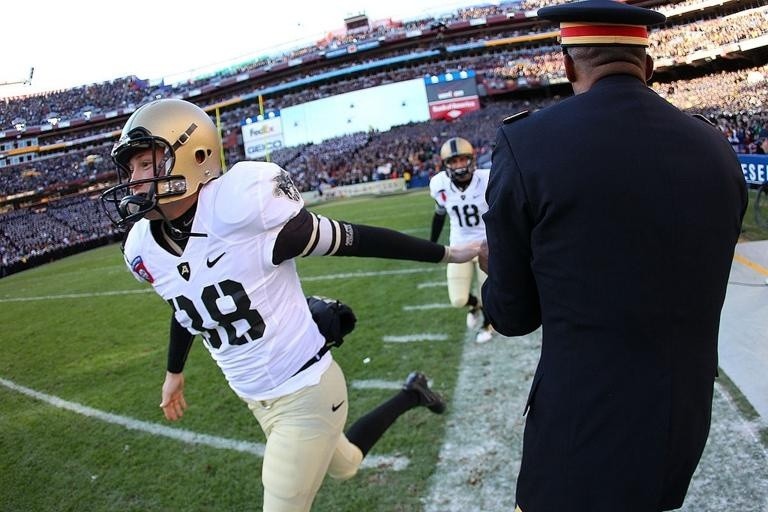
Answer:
403, 371, 448, 416
465, 297, 479, 331
474, 322, 497, 344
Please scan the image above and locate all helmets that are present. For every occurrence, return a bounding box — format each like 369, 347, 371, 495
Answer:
111, 98, 223, 204
438, 136, 475, 161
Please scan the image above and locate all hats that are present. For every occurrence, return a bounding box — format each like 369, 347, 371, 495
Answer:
537, 0, 667, 48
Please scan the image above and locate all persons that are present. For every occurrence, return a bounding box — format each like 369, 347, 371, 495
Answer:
430, 137, 494, 342
123, 98, 482, 512
479, 0, 749, 512
0, 1, 768, 268
